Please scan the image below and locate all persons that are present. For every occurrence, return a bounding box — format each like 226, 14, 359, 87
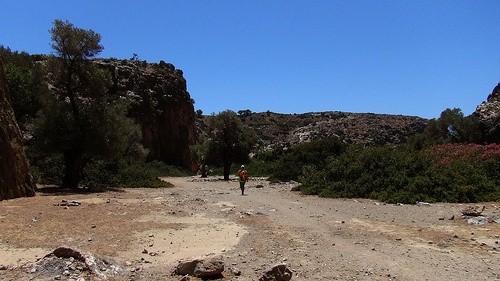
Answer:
236, 165, 248, 195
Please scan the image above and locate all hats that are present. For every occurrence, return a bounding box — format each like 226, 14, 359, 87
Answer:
241, 164, 245, 168
